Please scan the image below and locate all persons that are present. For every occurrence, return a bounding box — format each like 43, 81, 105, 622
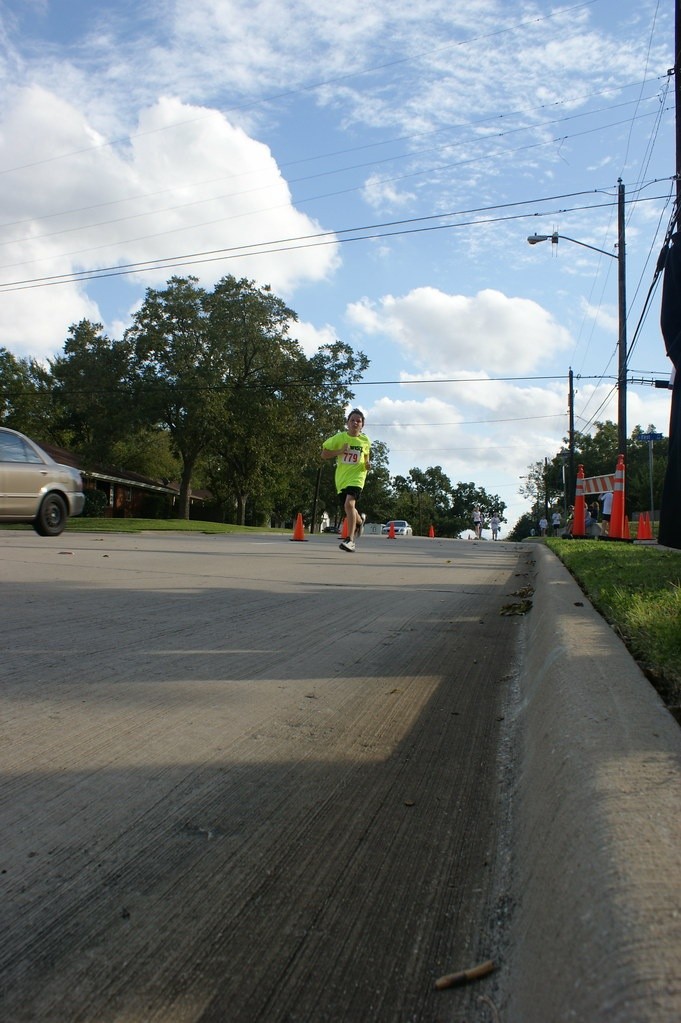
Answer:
471, 506, 501, 541
323, 410, 371, 552
539, 492, 614, 539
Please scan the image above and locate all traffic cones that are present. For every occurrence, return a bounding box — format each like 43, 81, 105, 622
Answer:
387, 522, 397, 539
562, 454, 654, 542
336, 515, 349, 539
289, 513, 310, 542
429, 524, 434, 538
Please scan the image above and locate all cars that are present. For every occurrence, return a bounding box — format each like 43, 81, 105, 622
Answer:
0, 426, 86, 537
382, 520, 413, 536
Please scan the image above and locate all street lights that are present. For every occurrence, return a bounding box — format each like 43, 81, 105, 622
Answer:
527, 234, 632, 524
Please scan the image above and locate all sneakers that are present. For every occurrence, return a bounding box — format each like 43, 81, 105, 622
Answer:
337, 537, 355, 552
354, 517, 364, 538
